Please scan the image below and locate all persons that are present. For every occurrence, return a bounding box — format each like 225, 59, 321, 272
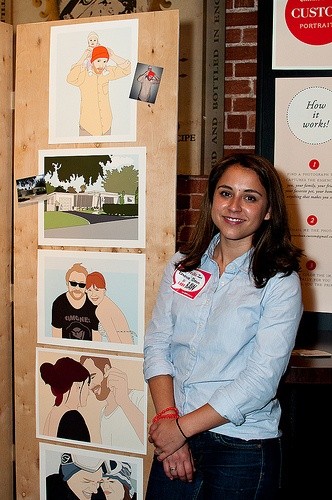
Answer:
142, 153, 305, 500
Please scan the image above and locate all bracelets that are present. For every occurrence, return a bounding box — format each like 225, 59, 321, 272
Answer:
175, 418, 190, 440
152, 407, 180, 424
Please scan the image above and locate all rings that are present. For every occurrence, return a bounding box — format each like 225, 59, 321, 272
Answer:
170, 466, 176, 472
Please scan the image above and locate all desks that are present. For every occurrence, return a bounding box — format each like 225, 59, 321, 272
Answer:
282, 330, 332, 431
